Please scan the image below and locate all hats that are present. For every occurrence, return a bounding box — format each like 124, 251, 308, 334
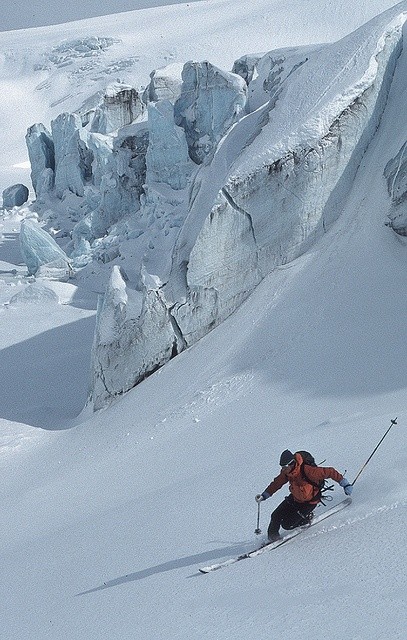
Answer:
280, 449, 293, 466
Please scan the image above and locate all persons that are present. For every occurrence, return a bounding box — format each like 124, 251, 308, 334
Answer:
254, 449, 355, 542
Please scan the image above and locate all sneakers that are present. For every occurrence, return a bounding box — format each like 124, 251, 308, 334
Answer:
303, 512, 313, 525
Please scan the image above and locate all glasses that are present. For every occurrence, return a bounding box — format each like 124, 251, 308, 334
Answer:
280, 465, 289, 469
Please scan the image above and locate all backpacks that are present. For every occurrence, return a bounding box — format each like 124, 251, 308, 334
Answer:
290, 449, 335, 507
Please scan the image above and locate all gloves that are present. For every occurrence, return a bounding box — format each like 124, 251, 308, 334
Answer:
255, 491, 270, 502
340, 478, 353, 496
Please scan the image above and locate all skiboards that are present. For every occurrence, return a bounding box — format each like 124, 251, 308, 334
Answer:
197, 497, 352, 574
